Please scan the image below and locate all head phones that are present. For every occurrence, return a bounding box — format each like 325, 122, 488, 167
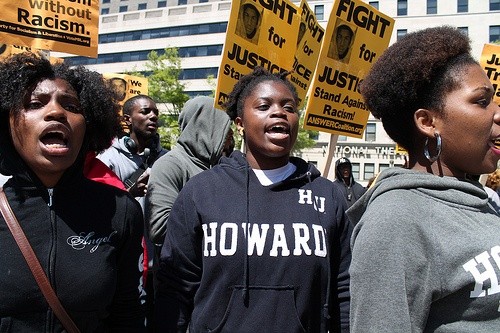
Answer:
125, 139, 156, 156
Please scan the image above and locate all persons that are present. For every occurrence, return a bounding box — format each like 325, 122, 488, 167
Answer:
0, 26, 500, 333
332, 20, 357, 64
110, 77, 127, 101
297, 22, 306, 44
241, 1, 264, 45
